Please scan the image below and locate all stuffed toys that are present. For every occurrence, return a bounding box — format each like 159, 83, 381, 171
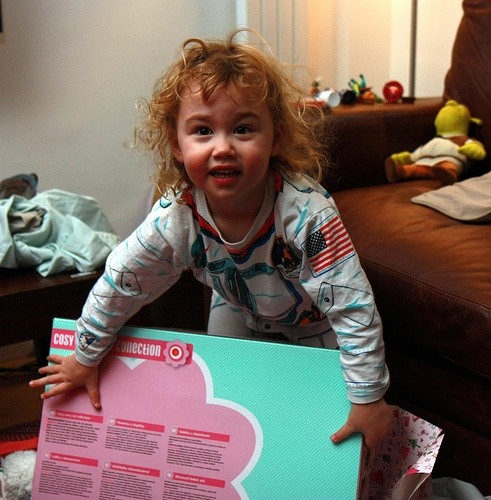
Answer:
385, 99, 487, 184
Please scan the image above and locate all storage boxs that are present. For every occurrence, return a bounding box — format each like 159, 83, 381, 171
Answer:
28, 317, 369, 500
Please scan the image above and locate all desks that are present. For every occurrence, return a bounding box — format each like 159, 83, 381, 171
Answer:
0, 266, 151, 377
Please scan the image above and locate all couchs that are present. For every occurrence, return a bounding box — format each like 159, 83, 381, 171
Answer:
296, 1, 491, 497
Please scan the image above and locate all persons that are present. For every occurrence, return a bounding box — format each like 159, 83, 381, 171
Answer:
28, 40, 399, 448
